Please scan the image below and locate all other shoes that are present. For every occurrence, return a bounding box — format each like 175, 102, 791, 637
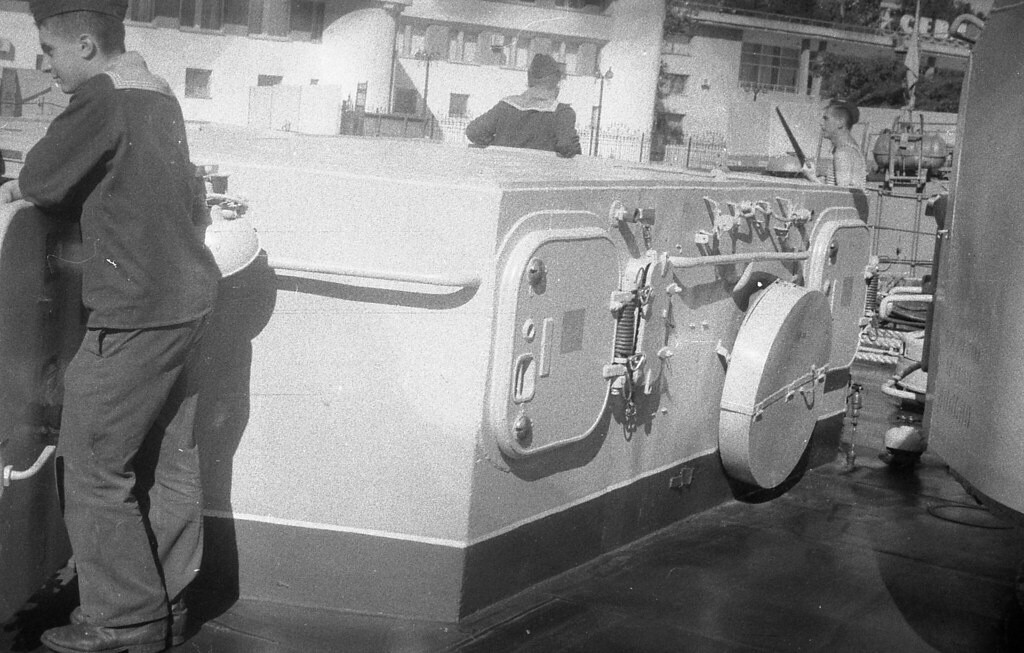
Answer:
40, 623, 167, 653
70, 604, 189, 646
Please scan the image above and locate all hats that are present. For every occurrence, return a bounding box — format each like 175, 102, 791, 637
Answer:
831, 98, 860, 124
28, 0, 129, 21
531, 54, 560, 78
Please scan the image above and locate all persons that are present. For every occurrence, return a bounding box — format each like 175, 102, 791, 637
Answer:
0, 0, 222, 653
466, 53, 582, 156
802, 100, 867, 188
